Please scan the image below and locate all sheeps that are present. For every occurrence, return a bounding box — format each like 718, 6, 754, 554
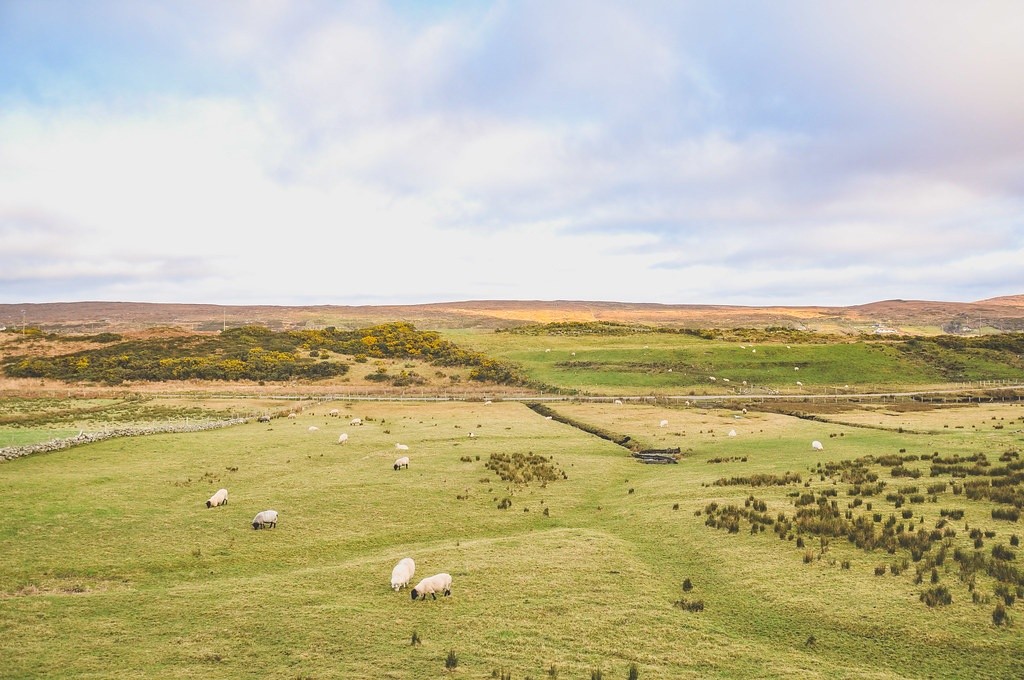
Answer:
393, 457, 409, 471
259, 415, 270, 423
729, 430, 736, 438
411, 574, 452, 602
615, 400, 622, 405
348, 419, 362, 426
252, 511, 278, 530
308, 424, 319, 431
205, 489, 228, 509
661, 420, 668, 428
482, 401, 491, 407
395, 442, 409, 450
287, 412, 296, 419
468, 432, 474, 437
390, 558, 415, 592
812, 441, 824, 451
743, 408, 747, 414
329, 409, 339, 417
338, 434, 347, 444
